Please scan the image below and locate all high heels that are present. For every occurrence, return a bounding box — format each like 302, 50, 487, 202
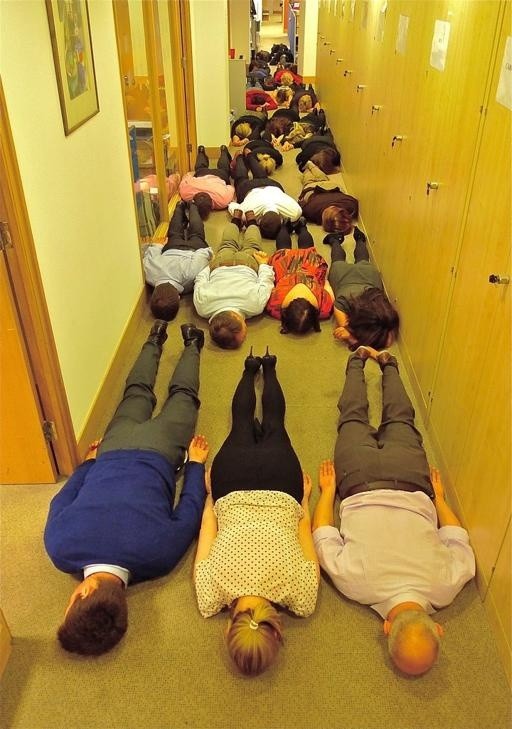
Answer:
323, 230, 344, 245
354, 226, 365, 242
245, 346, 262, 373
261, 345, 277, 367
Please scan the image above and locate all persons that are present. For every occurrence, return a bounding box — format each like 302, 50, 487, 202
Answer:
175, 144, 236, 220
135, 164, 182, 213
142, 198, 215, 321
43, 318, 210, 659
323, 225, 401, 352
310, 343, 477, 680
297, 159, 360, 235
190, 344, 322, 679
192, 206, 276, 352
265, 216, 335, 338
227, 149, 304, 239
230, 43, 342, 176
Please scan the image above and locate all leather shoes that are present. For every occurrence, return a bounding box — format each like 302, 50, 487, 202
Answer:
245, 210, 256, 227
230, 148, 251, 178
283, 217, 293, 233
233, 209, 244, 228
198, 146, 209, 167
293, 216, 306, 231
221, 145, 231, 161
180, 323, 204, 347
176, 200, 199, 229
150, 319, 168, 345
299, 157, 309, 173
345, 345, 371, 376
376, 350, 399, 375
313, 108, 324, 116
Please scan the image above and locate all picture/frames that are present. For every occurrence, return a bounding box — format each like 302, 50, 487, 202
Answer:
43, 0, 100, 136
